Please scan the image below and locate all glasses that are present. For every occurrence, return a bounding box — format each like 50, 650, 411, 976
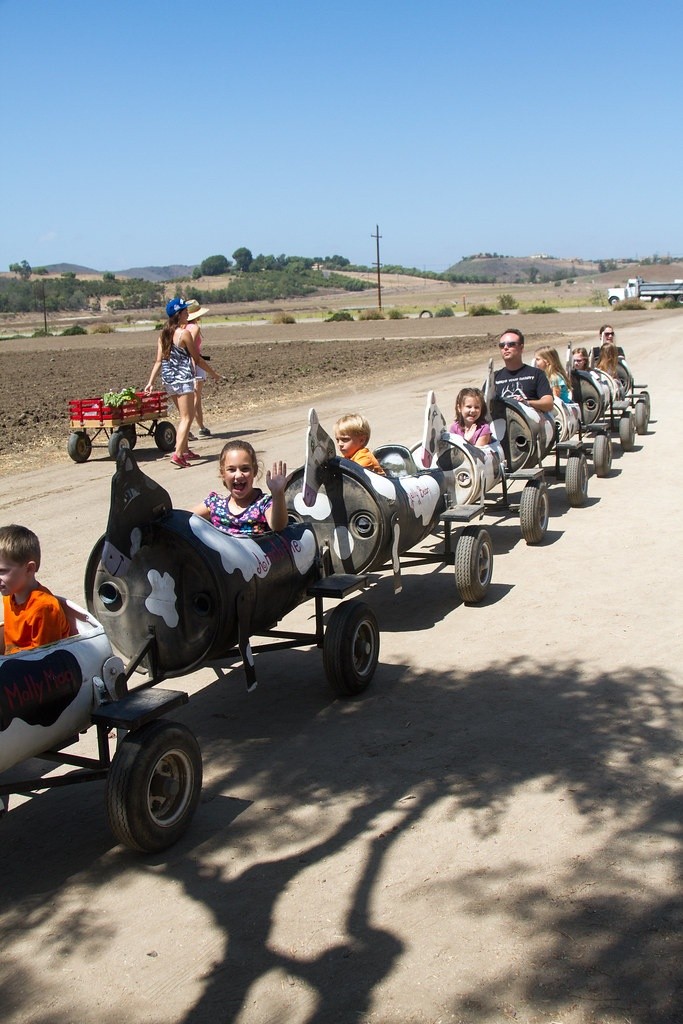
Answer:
604, 332, 614, 336
499, 342, 520, 347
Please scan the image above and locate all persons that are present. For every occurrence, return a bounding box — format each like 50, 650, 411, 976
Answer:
596, 343, 619, 379
534, 346, 573, 403
572, 348, 589, 370
181, 300, 209, 440
589, 325, 625, 368
450, 388, 493, 446
334, 415, 388, 476
144, 298, 229, 467
196, 440, 293, 538
0, 524, 70, 655
482, 328, 554, 411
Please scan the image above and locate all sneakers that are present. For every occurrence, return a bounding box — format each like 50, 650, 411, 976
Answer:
188, 432, 198, 441
170, 454, 191, 468
198, 428, 210, 437
182, 450, 200, 460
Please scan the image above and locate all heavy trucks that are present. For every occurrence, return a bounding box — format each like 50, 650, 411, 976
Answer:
608, 276, 683, 305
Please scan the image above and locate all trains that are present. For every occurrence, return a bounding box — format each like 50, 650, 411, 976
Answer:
0, 344, 650, 850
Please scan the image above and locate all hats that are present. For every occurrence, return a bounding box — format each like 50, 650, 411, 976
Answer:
186, 300, 210, 321
166, 298, 193, 315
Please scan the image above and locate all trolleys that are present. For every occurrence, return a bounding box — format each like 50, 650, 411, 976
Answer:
67, 386, 177, 462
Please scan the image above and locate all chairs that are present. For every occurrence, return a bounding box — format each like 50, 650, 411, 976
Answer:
372, 445, 418, 475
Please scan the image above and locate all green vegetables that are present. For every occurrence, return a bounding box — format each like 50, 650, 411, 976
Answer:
104, 386, 141, 408
